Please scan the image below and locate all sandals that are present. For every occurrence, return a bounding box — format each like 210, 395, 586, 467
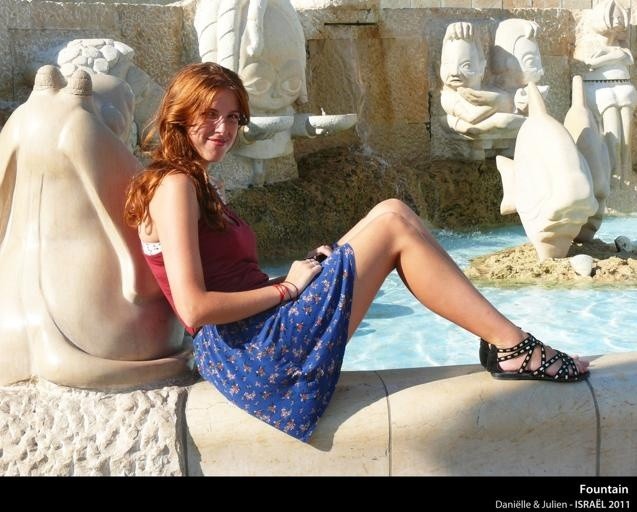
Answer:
480, 332, 590, 382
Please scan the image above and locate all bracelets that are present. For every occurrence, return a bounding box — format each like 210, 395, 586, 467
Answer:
274, 280, 300, 303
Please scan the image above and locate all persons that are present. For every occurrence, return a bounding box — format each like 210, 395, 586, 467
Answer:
584, 0, 637, 189
124, 62, 590, 442
490, 18, 550, 116
193, 1, 336, 191
439, 22, 523, 136
